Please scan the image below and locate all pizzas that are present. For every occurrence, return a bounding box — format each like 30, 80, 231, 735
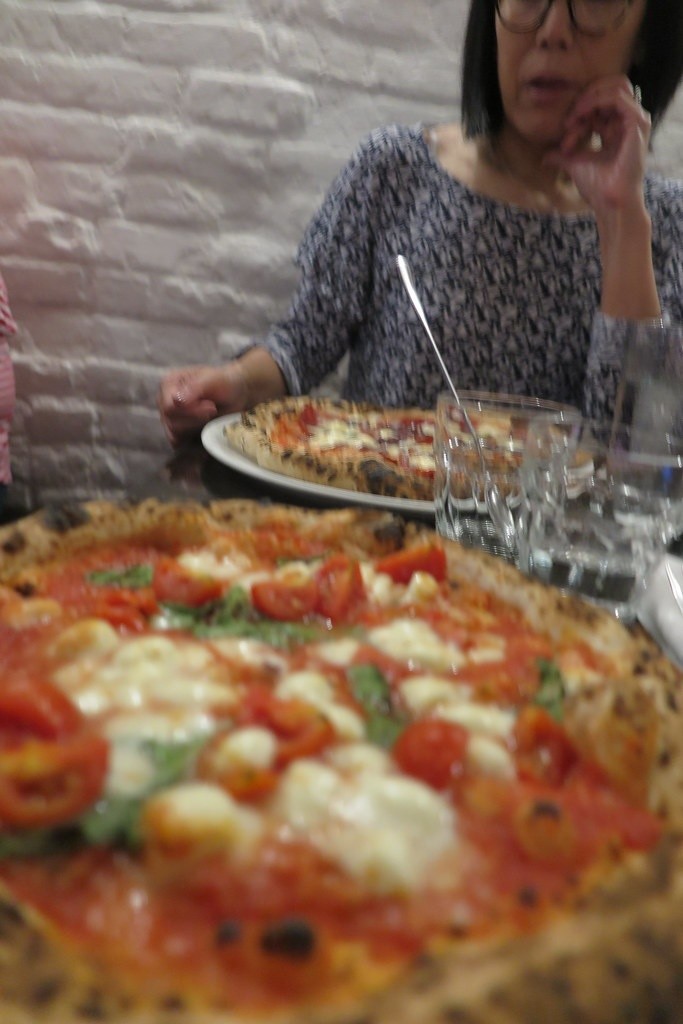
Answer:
0, 496, 683, 1024
223, 398, 588, 502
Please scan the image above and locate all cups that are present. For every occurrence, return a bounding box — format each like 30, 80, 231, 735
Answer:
435, 391, 582, 567
608, 316, 683, 452
519, 412, 682, 626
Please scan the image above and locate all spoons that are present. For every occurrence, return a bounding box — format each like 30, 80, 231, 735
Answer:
396, 254, 516, 547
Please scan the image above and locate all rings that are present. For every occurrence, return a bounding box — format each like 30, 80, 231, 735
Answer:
634, 85, 642, 103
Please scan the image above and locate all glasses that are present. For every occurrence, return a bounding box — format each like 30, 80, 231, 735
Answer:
493, 0, 633, 39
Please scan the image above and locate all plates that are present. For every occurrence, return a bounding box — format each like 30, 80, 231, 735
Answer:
201, 412, 434, 511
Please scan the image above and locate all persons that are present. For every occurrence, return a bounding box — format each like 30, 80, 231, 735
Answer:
0, 277, 17, 493
157, 0, 683, 463
